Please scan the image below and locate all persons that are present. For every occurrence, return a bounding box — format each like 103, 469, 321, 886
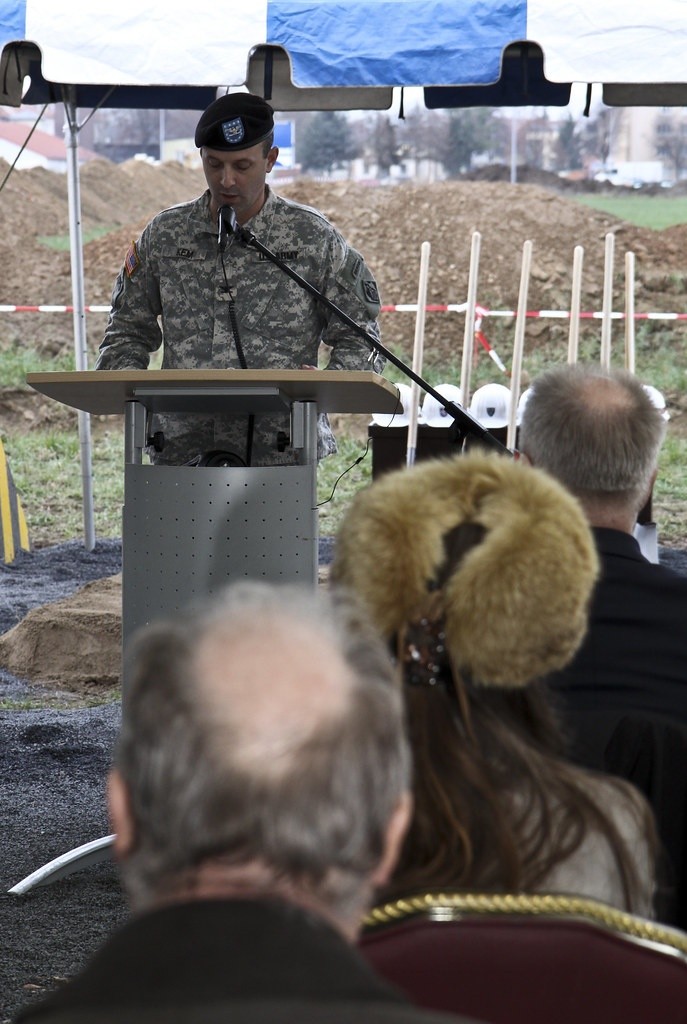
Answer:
97, 94, 380, 469
327, 363, 687, 931
12, 579, 476, 1024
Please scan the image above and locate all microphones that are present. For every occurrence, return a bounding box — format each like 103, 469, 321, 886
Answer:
216, 204, 235, 249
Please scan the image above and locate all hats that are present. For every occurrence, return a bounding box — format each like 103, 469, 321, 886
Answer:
194, 93, 274, 151
328, 446, 599, 688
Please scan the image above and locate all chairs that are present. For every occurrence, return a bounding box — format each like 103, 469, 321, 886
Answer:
357, 894, 687, 1024
